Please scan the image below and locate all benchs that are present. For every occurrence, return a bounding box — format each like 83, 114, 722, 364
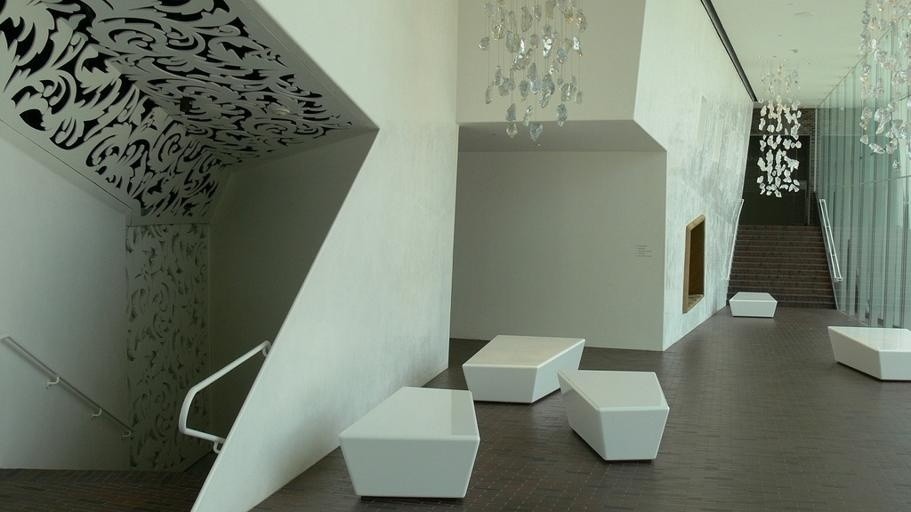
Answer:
726, 288, 781, 319
330, 383, 486, 501
555, 363, 673, 464
828, 324, 911, 383
460, 332, 591, 405
